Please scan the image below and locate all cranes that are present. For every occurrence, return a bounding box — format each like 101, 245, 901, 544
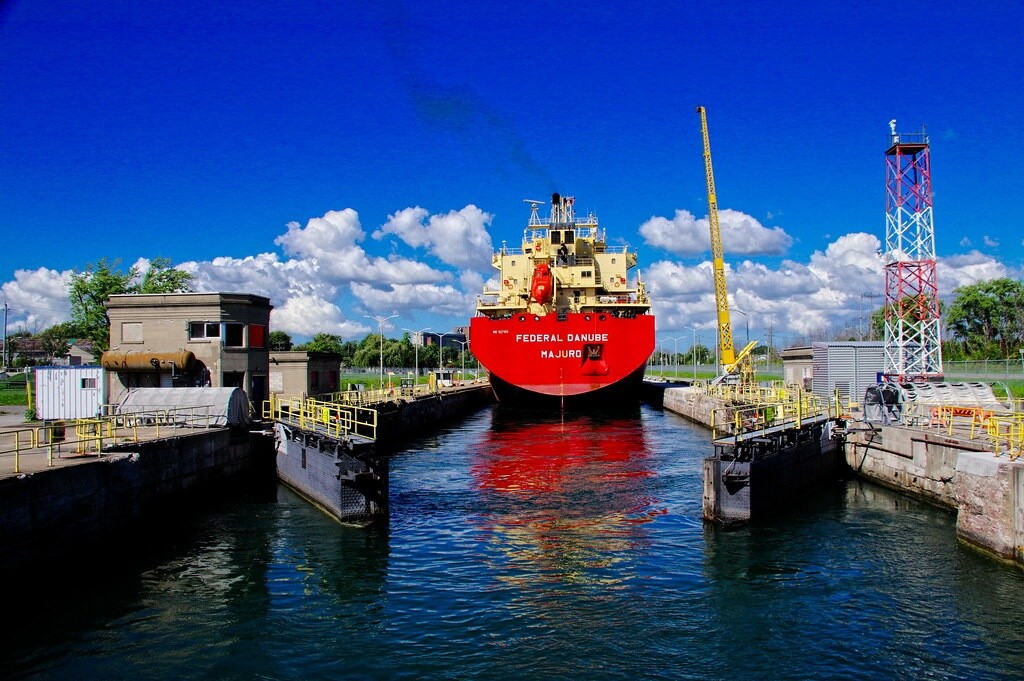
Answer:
697, 106, 759, 404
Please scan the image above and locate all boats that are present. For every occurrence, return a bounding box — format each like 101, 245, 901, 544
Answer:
468, 193, 655, 406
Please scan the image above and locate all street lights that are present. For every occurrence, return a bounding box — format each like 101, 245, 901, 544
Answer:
400, 328, 431, 386
656, 336, 671, 381
729, 307, 762, 346
667, 336, 686, 381
427, 331, 457, 381
452, 339, 471, 385
363, 315, 400, 389
685, 325, 707, 385
705, 322, 721, 380
